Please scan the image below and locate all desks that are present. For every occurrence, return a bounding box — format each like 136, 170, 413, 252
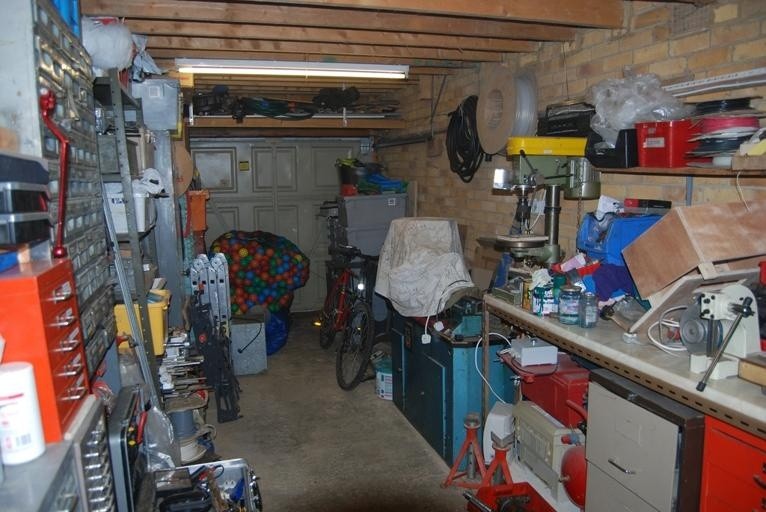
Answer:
481, 290, 766, 512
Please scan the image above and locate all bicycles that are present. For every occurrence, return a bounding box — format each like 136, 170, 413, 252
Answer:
317, 243, 378, 391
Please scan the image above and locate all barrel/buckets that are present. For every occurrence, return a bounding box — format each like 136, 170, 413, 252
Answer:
169, 409, 198, 442
374, 357, 393, 402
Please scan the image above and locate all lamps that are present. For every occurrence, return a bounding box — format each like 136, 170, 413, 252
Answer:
173, 55, 411, 81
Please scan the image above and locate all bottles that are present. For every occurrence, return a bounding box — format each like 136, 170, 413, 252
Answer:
557, 284, 582, 326
577, 293, 599, 327
0, 361, 46, 465
552, 274, 567, 305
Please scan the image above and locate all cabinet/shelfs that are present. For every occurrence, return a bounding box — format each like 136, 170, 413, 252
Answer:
698, 410, 766, 512
1, 0, 126, 512
91, 65, 183, 430
378, 292, 523, 476
577, 362, 706, 512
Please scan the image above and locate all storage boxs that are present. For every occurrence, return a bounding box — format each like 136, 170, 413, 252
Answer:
335, 190, 407, 228
335, 221, 389, 257
635, 118, 713, 170
